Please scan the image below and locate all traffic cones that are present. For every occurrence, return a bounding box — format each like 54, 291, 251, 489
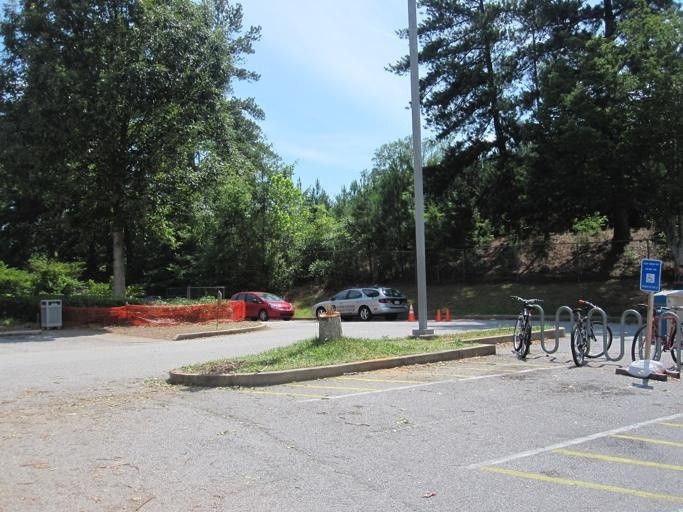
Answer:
407, 303, 416, 321
436, 309, 442, 322
445, 309, 451, 321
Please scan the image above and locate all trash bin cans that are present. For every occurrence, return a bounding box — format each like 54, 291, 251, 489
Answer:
40, 299, 63, 330
654, 289, 683, 345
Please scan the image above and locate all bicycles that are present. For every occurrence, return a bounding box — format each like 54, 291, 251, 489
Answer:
509, 294, 545, 357
631, 304, 683, 366
570, 300, 613, 367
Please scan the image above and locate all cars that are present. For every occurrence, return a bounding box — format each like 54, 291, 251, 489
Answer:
310, 287, 408, 321
227, 292, 294, 321
